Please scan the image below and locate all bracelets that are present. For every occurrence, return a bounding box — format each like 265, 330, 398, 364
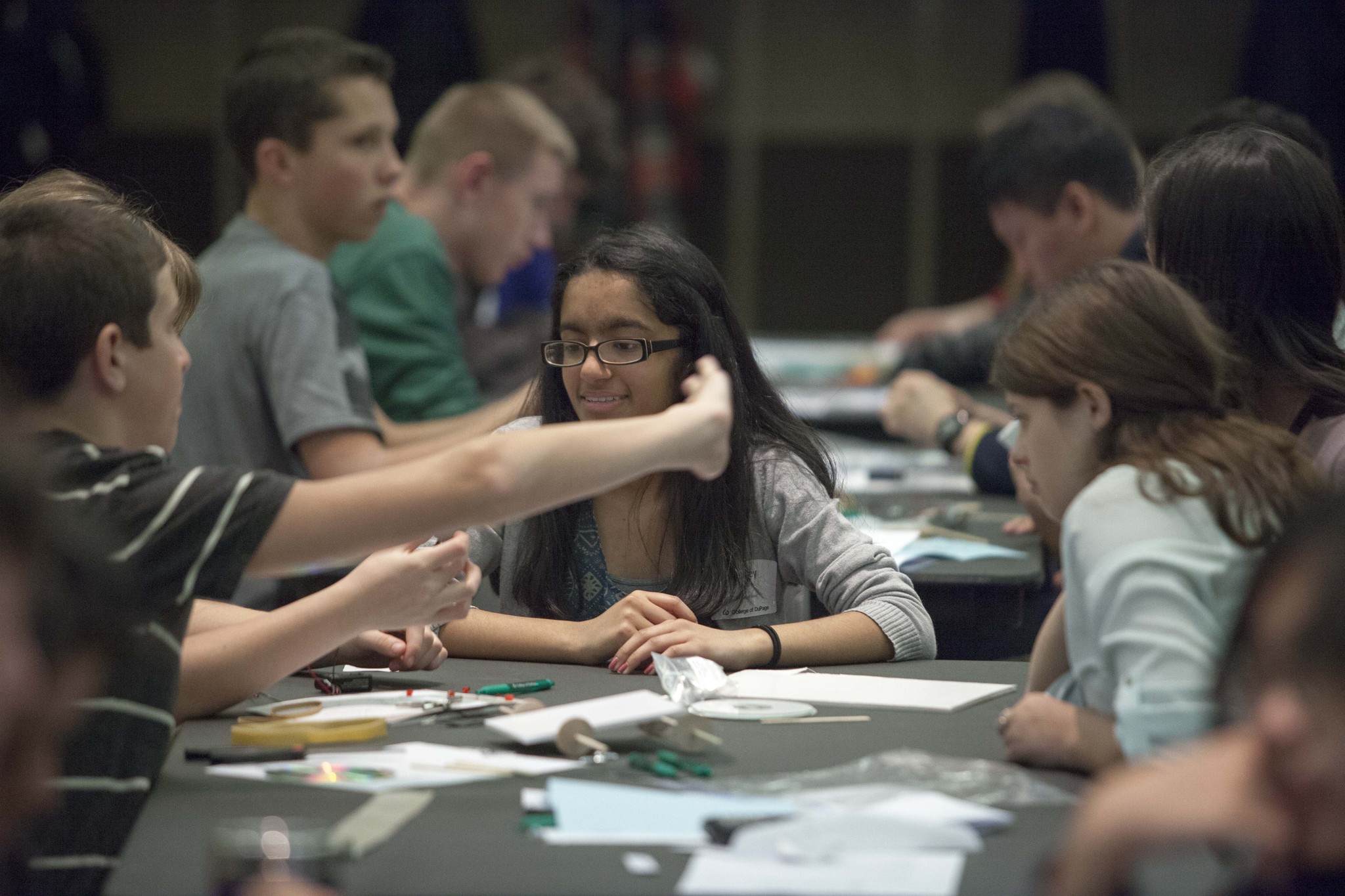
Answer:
751, 624, 781, 666
936, 410, 971, 454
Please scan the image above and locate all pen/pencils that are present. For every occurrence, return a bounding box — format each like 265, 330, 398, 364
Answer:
474, 678, 556, 696
628, 750, 676, 777
656, 750, 711, 777
185, 745, 309, 764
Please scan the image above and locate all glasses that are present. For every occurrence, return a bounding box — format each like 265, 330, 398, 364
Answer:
541, 337, 685, 368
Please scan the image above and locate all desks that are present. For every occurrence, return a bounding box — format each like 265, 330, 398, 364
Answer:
92, 656, 1078, 896
810, 434, 1045, 660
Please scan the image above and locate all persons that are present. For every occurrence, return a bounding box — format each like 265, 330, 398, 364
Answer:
417, 224, 941, 672
875, 268, 1013, 342
881, 74, 1150, 502
0, 169, 732, 896
1024, 486, 1345, 896
989, 259, 1327, 896
1012, 128, 1345, 593
0, 423, 335, 896
325, 83, 573, 581
1171, 102, 1326, 168
482, 51, 620, 330
161, 28, 534, 615
